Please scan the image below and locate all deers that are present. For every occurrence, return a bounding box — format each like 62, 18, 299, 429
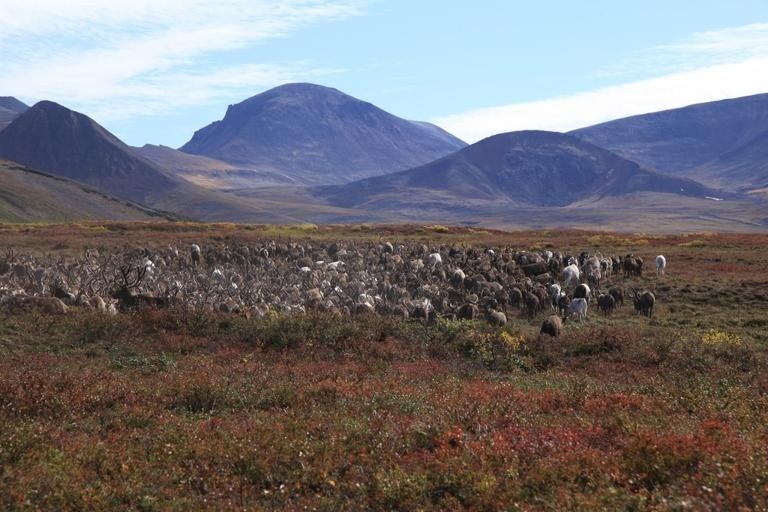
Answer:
1, 230, 669, 339
